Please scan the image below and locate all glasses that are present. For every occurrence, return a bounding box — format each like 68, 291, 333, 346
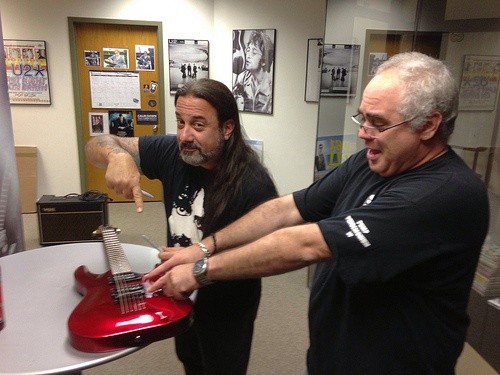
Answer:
351, 113, 415, 138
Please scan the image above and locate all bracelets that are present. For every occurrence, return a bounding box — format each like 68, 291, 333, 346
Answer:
210, 233, 217, 253
196, 242, 211, 256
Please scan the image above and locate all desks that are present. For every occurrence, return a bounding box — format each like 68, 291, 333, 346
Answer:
0, 242, 199, 375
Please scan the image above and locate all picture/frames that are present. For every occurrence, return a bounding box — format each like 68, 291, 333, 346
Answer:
2, 38, 53, 106
304, 36, 325, 102
169, 39, 209, 94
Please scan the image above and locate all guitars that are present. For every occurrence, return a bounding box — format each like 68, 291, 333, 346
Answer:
67, 224, 197, 353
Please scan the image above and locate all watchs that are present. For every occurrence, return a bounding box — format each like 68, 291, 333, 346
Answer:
193, 257, 212, 284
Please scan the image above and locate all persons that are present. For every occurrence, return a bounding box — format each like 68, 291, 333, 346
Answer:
146, 52, 490, 375
9, 49, 45, 59
181, 63, 197, 78
331, 67, 346, 81
97, 114, 132, 137
89, 50, 149, 67
86, 78, 278, 375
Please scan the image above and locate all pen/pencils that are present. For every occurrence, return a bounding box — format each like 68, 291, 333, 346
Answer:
142, 234, 163, 251
141, 190, 155, 199
